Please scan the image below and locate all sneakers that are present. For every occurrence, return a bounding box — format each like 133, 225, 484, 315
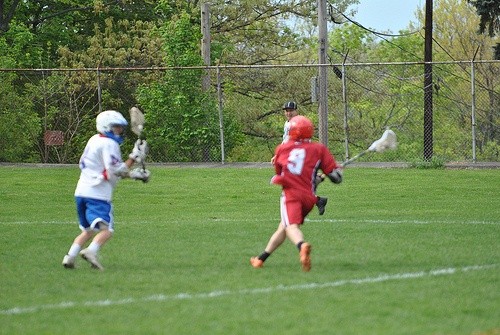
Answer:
79, 248, 104, 270
316, 197, 328, 214
62, 254, 76, 268
249, 255, 263, 269
300, 242, 310, 271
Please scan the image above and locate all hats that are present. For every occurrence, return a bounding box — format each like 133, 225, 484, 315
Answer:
96, 110, 128, 144
281, 101, 298, 110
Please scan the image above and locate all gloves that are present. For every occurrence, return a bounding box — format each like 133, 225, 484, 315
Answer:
129, 168, 151, 181
129, 139, 148, 164
316, 174, 325, 183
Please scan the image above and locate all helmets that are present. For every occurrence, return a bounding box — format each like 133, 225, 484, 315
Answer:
287, 115, 315, 142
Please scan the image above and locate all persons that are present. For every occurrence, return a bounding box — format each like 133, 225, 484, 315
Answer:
249, 116, 343, 271
270, 101, 328, 214
62, 110, 152, 269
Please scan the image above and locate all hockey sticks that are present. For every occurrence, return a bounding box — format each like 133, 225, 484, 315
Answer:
130, 107, 148, 183
316, 130, 397, 186
